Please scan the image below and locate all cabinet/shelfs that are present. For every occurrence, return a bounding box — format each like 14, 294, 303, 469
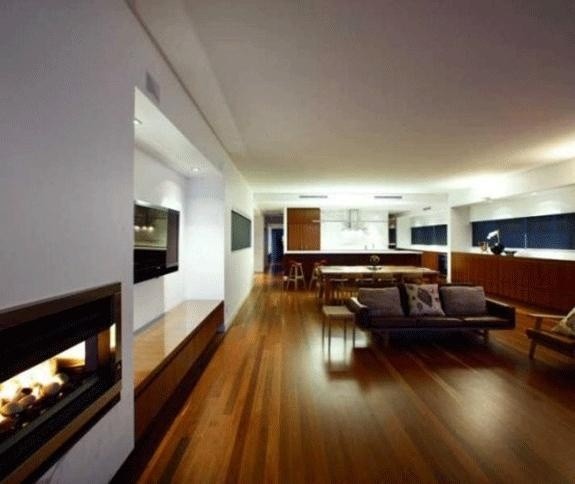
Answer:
288, 208, 320, 250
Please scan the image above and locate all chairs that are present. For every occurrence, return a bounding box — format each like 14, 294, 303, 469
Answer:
370, 273, 394, 287
524, 312, 575, 361
400, 273, 423, 284
336, 272, 364, 306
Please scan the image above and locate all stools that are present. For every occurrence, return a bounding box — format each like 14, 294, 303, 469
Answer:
321, 306, 357, 344
286, 262, 306, 291
309, 267, 318, 291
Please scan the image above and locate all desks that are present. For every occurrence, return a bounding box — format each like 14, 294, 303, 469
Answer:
318, 265, 439, 303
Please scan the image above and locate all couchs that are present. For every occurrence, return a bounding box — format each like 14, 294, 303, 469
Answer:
345, 296, 516, 350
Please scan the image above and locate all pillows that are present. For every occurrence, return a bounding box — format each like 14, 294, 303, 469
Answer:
550, 308, 575, 339
358, 281, 488, 316
